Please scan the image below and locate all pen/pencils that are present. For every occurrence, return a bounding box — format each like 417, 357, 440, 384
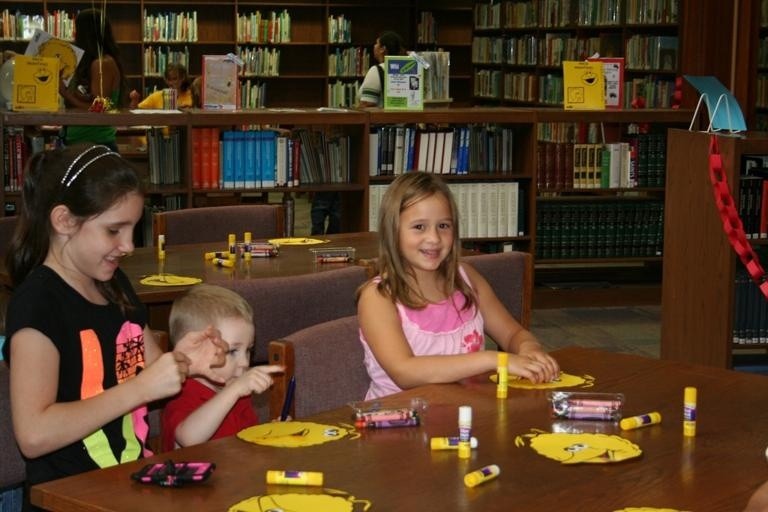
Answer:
280, 376, 296, 421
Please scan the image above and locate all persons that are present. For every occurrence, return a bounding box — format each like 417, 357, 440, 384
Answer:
355, 172, 560, 403
2, 143, 229, 512
129, 62, 203, 109
157, 284, 286, 452
355, 31, 403, 109
59, 9, 124, 154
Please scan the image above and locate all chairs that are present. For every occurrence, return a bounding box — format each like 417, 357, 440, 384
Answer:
266, 316, 371, 422
1, 330, 168, 491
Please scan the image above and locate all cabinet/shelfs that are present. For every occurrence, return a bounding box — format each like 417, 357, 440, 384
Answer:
0, 0, 767, 375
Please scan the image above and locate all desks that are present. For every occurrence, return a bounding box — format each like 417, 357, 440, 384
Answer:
31, 344, 768, 512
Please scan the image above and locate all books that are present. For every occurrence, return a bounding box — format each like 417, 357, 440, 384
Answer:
2, 8, 86, 113
237, 8, 291, 109
143, 7, 198, 99
472, 1, 680, 109
328, 14, 370, 108
406, 11, 450, 100
731, 0, 768, 373
3, 122, 682, 260
